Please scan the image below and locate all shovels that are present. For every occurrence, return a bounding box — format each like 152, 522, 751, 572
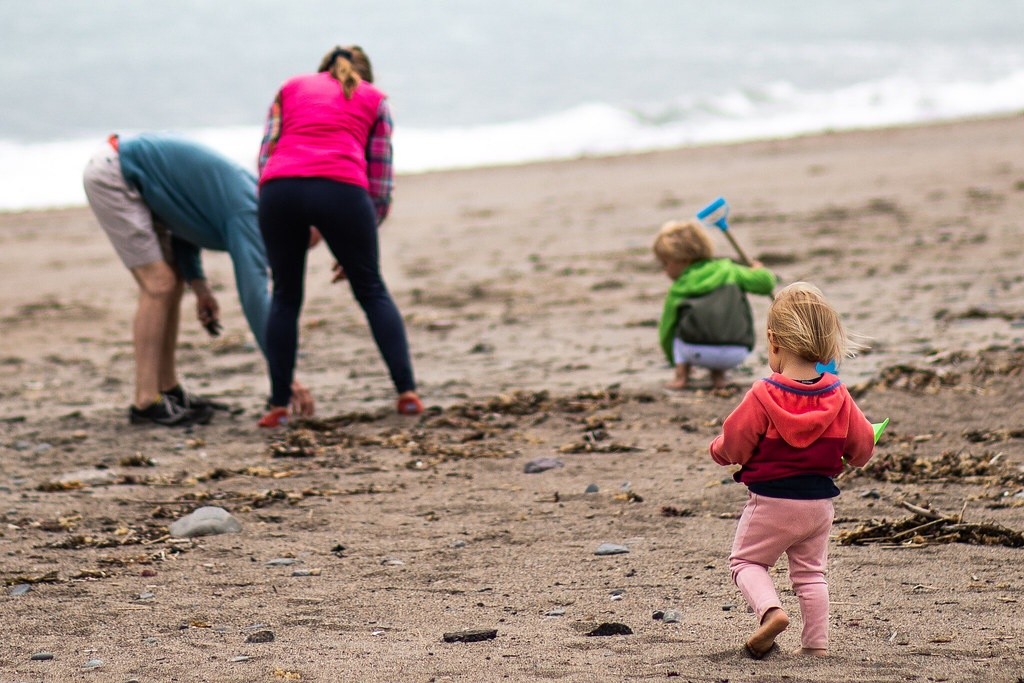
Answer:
697, 197, 776, 299
838, 416, 888, 468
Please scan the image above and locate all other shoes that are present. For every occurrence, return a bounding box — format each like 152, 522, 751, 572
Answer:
397, 395, 424, 416
259, 410, 290, 426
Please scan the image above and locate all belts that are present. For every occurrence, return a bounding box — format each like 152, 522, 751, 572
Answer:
108, 133, 121, 152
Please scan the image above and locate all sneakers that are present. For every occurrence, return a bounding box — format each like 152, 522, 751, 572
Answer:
160, 385, 215, 424
128, 395, 196, 428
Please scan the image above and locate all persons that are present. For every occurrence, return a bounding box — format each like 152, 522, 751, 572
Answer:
257, 46, 425, 429
652, 219, 777, 399
83, 131, 325, 429
709, 281, 875, 657
